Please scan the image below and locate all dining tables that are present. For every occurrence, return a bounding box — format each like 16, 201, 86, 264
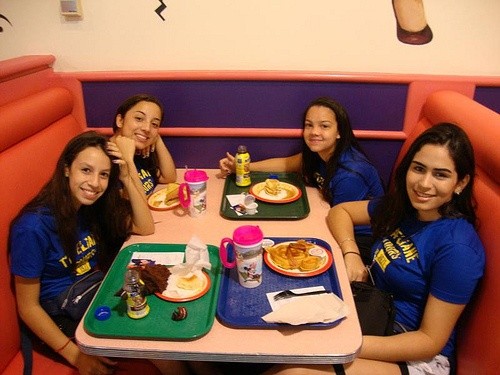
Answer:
74, 168, 364, 375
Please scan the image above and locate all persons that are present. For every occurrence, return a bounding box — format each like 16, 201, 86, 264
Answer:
220, 97, 388, 265
108, 93, 177, 200
267, 124, 486, 375
8, 130, 212, 375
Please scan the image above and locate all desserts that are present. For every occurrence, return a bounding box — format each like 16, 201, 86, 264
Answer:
141, 263, 172, 293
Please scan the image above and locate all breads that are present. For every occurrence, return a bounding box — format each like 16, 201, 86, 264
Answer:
266, 240, 321, 270
265, 179, 281, 195
177, 275, 199, 290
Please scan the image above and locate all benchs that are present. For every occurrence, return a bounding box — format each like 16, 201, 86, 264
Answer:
0, 85, 163, 375
387, 90, 500, 375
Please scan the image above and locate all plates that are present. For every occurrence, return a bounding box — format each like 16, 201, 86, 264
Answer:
249, 181, 302, 204
147, 184, 180, 211
264, 240, 333, 278
155, 266, 211, 303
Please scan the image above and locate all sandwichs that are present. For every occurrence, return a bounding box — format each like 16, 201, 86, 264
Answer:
166, 182, 185, 206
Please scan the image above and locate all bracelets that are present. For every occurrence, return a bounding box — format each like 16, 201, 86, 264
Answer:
343, 252, 361, 257
55, 338, 71, 352
340, 238, 356, 248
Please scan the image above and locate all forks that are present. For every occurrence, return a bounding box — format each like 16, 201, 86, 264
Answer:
274, 289, 331, 302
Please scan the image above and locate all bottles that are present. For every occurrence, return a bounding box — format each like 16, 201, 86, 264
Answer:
235, 145, 251, 188
219, 225, 264, 289
178, 170, 209, 217
124, 264, 150, 319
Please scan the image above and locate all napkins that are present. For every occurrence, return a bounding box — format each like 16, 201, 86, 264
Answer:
184, 237, 212, 271
129, 251, 184, 266
261, 285, 351, 325
225, 194, 258, 217
